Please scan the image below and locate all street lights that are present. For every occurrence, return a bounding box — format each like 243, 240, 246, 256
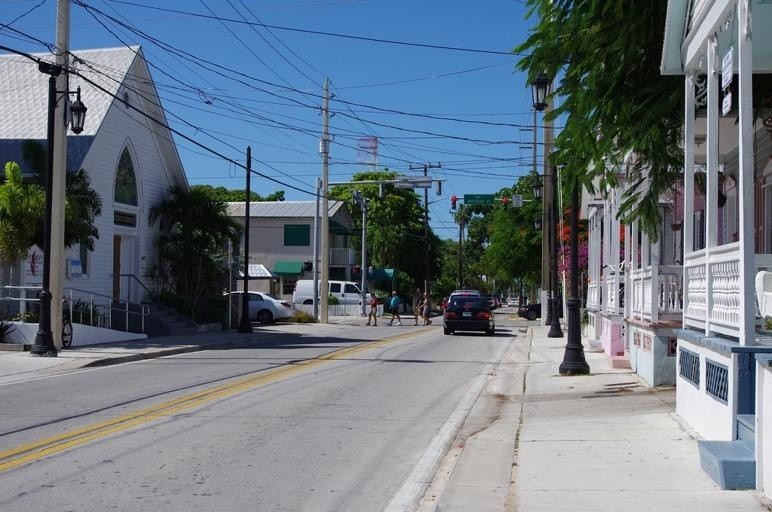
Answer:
534, 203, 553, 324
28, 73, 88, 354
529, 65, 593, 375
531, 165, 565, 338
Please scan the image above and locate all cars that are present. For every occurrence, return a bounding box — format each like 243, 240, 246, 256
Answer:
442, 289, 521, 336
519, 297, 563, 321
223, 291, 294, 325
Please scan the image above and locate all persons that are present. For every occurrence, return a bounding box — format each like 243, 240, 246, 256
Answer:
412, 288, 424, 325
366, 293, 378, 326
419, 293, 432, 326
386, 290, 402, 326
440, 297, 447, 308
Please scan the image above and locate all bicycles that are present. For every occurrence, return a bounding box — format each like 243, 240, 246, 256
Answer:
600, 260, 625, 307
60, 298, 73, 348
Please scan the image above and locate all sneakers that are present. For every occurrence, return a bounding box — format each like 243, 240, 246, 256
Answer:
366, 321, 432, 326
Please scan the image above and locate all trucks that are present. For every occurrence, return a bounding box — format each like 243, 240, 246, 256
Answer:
292, 280, 377, 306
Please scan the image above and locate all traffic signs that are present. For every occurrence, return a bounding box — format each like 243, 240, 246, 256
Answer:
464, 194, 493, 203
513, 195, 522, 207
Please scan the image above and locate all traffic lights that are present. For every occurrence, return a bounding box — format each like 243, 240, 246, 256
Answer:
451, 195, 456, 209
501, 196, 509, 211
